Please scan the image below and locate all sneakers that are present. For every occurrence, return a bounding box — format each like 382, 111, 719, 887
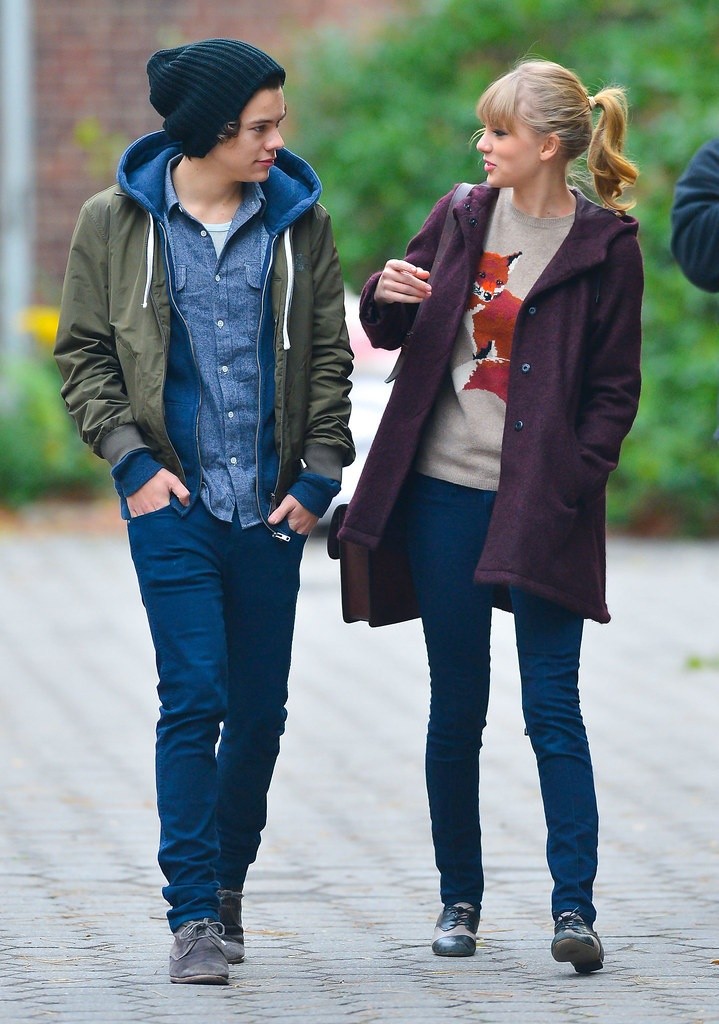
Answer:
432, 902, 481, 956
550, 907, 604, 973
217, 884, 245, 964
169, 918, 229, 984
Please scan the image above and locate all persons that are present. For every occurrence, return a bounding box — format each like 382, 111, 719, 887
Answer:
669, 133, 719, 296
336, 59, 647, 977
53, 36, 357, 988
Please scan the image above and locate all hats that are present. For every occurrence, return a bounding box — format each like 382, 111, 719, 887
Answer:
146, 38, 286, 157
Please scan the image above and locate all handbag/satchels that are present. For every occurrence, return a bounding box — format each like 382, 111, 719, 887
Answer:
327, 503, 422, 628
